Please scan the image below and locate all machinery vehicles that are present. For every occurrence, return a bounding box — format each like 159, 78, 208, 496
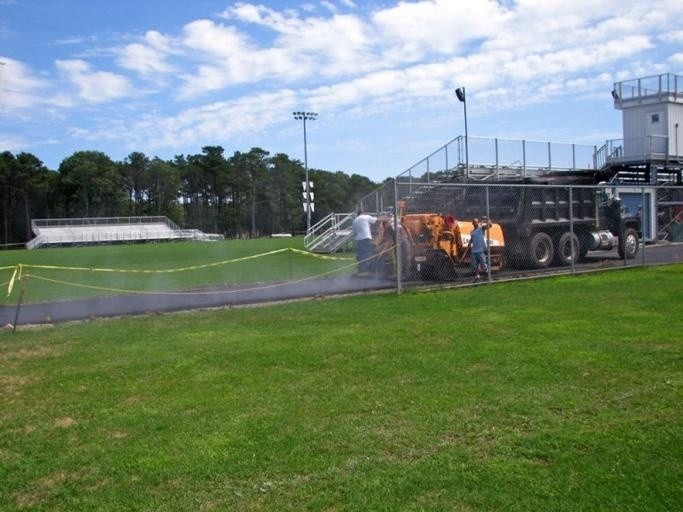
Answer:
371, 200, 505, 278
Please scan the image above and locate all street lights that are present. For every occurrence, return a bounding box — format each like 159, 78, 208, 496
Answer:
455, 87, 468, 183
292, 111, 318, 237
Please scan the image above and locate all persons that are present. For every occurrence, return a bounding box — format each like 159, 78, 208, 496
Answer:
353, 210, 377, 274
382, 221, 415, 280
468, 215, 493, 280
